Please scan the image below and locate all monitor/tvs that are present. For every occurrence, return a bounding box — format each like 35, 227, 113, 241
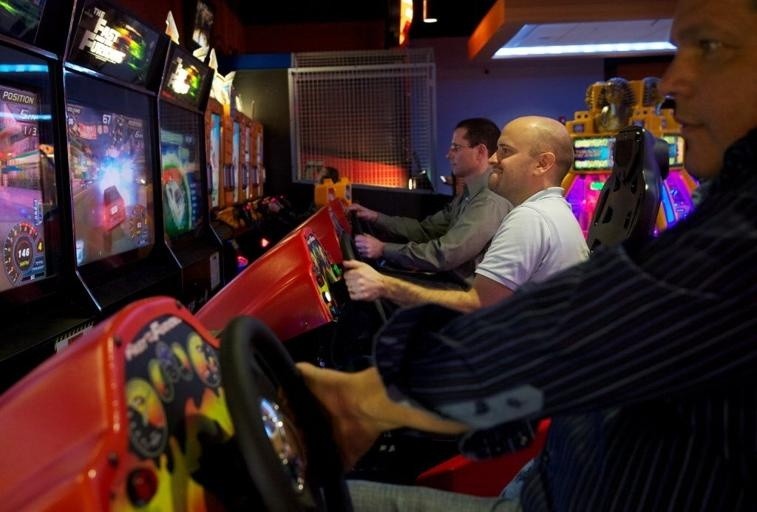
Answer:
0, 86, 47, 293
65, 103, 156, 266
663, 135, 684, 166
210, 112, 221, 208
573, 135, 614, 171
160, 124, 202, 242
231, 121, 263, 203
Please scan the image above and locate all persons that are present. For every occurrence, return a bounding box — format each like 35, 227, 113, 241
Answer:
343, 114, 591, 315
346, 116, 514, 274
295, 0, 757, 512
